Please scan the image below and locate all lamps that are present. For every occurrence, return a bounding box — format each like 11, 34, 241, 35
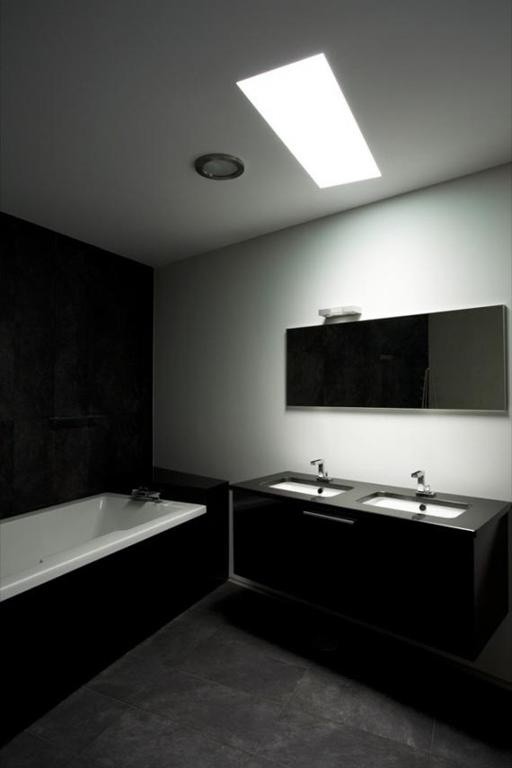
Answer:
196, 153, 243, 179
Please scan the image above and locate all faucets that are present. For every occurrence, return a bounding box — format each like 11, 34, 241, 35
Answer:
309, 458, 332, 481
131, 488, 161, 505
411, 471, 435, 497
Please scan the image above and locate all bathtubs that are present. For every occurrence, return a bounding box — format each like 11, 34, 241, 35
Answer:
0, 492, 208, 602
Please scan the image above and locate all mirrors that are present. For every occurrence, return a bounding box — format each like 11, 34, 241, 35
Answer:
286, 313, 430, 408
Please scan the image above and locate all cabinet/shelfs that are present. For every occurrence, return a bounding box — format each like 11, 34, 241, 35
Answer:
284, 304, 510, 416
231, 492, 511, 661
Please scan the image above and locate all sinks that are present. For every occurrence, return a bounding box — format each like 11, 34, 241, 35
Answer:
259, 476, 354, 498
356, 491, 472, 518
